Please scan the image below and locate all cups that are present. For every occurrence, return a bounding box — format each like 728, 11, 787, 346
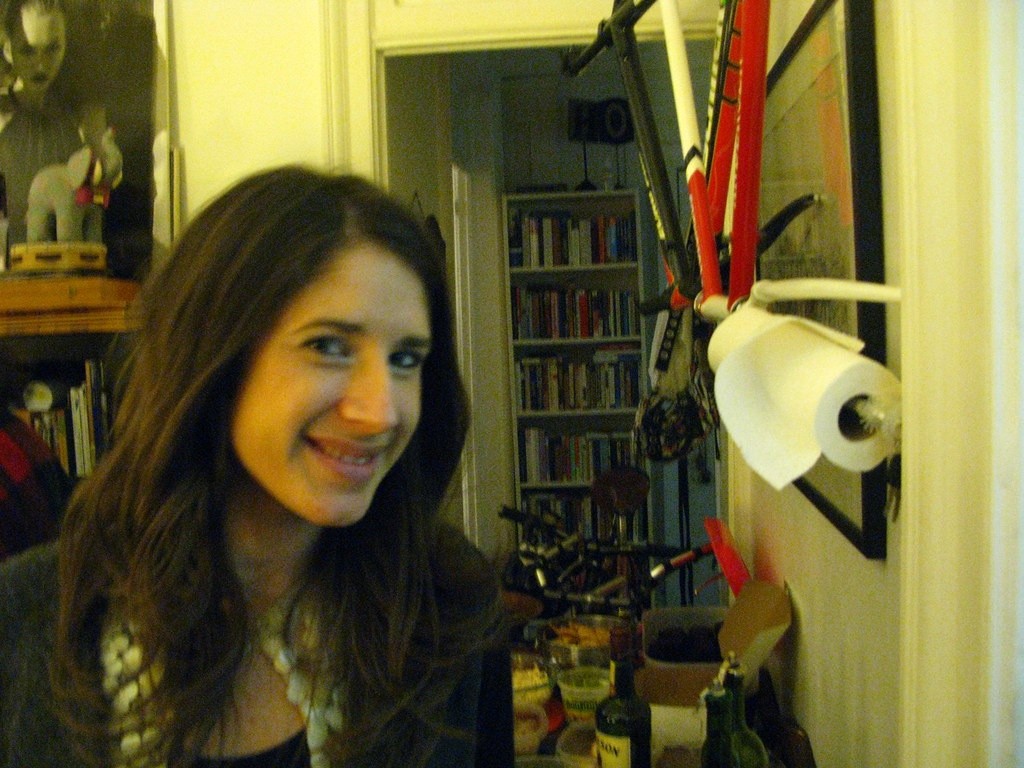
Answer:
556, 667, 610, 729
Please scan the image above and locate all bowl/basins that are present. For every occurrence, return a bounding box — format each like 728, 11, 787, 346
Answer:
538, 615, 626, 677
514, 670, 553, 706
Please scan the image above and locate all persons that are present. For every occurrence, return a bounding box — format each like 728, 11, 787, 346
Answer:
0, 0, 93, 243
0, 165, 516, 768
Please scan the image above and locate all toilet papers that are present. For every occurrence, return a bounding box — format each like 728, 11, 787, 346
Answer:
707, 305, 901, 495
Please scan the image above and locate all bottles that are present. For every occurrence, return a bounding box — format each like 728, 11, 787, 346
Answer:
701, 685, 740, 768
595, 657, 651, 768
722, 670, 769, 768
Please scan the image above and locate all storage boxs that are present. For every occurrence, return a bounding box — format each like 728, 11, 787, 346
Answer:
641, 577, 792, 706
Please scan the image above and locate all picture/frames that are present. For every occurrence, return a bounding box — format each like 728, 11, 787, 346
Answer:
758, 0, 888, 564
0, 0, 170, 283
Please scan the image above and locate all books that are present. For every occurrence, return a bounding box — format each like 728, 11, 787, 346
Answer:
522, 493, 647, 543
8, 358, 110, 477
514, 346, 641, 411
516, 428, 642, 484
509, 212, 637, 268
510, 284, 641, 341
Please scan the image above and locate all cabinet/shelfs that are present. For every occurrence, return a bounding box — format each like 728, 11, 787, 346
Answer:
501, 190, 667, 623
